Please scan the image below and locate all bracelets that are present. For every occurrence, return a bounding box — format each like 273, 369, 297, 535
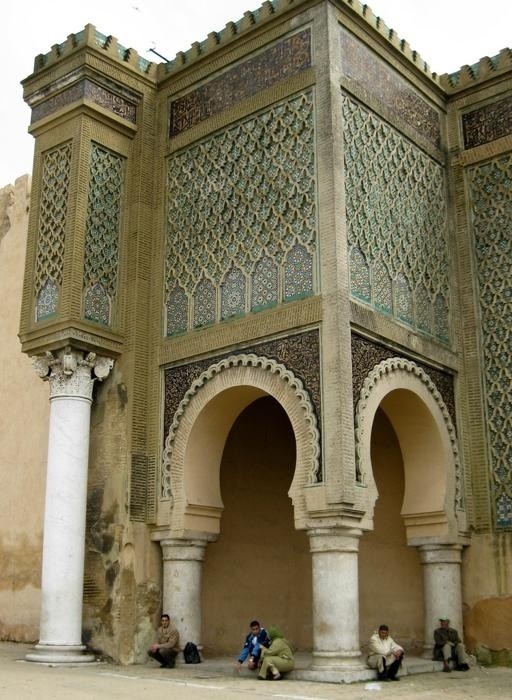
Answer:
248, 658, 254, 662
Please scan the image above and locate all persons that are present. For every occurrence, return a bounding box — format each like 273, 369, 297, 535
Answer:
365, 623, 406, 682
146, 614, 181, 669
235, 620, 273, 676
257, 624, 298, 680
432, 614, 469, 673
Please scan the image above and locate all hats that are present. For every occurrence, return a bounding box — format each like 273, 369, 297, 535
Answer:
438, 615, 450, 622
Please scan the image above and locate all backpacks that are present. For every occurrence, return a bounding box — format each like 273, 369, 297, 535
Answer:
182, 641, 201, 663
